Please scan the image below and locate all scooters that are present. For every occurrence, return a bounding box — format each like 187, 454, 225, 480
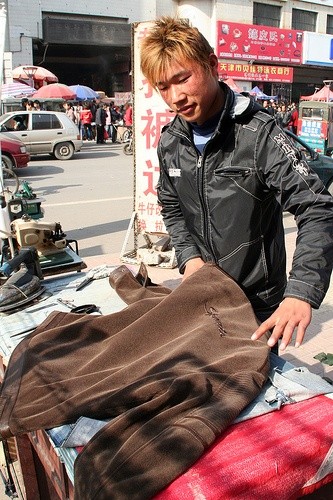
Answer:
121, 128, 135, 156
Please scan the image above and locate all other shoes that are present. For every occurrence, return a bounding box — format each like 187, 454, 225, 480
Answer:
97, 140, 106, 144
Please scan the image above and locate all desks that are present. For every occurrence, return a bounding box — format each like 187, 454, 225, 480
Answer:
0, 270, 333, 500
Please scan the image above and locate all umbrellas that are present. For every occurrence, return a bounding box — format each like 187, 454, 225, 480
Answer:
12, 66, 58, 87
32, 83, 76, 99
69, 84, 100, 101
0, 83, 36, 101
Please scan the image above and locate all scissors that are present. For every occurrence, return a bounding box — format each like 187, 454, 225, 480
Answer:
76, 267, 109, 291
10, 304, 96, 338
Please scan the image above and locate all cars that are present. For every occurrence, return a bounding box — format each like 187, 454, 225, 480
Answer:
0, 132, 31, 179
0, 110, 85, 161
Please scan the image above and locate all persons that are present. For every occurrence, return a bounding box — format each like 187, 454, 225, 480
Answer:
3, 98, 45, 130
263, 99, 298, 133
141, 19, 333, 355
63, 99, 132, 144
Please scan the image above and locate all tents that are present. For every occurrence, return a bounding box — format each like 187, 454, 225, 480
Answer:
223, 78, 257, 101
250, 86, 278, 104
300, 85, 333, 102
